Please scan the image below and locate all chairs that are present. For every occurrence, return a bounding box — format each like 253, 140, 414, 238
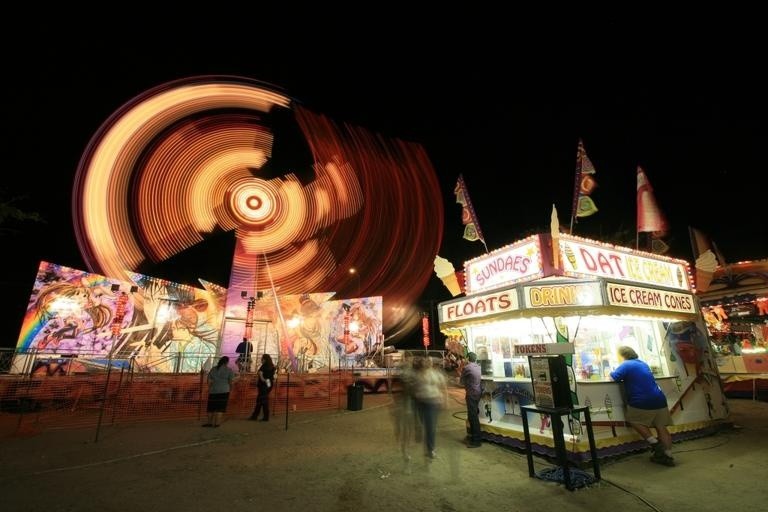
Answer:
240, 354, 251, 372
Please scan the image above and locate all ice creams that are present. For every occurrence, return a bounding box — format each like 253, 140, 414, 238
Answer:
564, 242, 579, 270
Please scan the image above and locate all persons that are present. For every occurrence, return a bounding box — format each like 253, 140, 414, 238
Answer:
609, 345, 674, 466
247, 354, 275, 421
38, 282, 113, 352
235, 337, 253, 372
389, 352, 481, 467
135, 310, 216, 373
337, 303, 371, 354
202, 356, 233, 428
143, 277, 195, 326
287, 294, 322, 360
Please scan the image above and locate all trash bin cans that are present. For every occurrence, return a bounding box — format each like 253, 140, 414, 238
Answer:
347, 384, 364, 411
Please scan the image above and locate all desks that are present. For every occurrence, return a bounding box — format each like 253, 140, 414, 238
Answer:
519, 403, 602, 489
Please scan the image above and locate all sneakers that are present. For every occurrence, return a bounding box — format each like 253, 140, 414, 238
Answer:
649, 440, 672, 465
